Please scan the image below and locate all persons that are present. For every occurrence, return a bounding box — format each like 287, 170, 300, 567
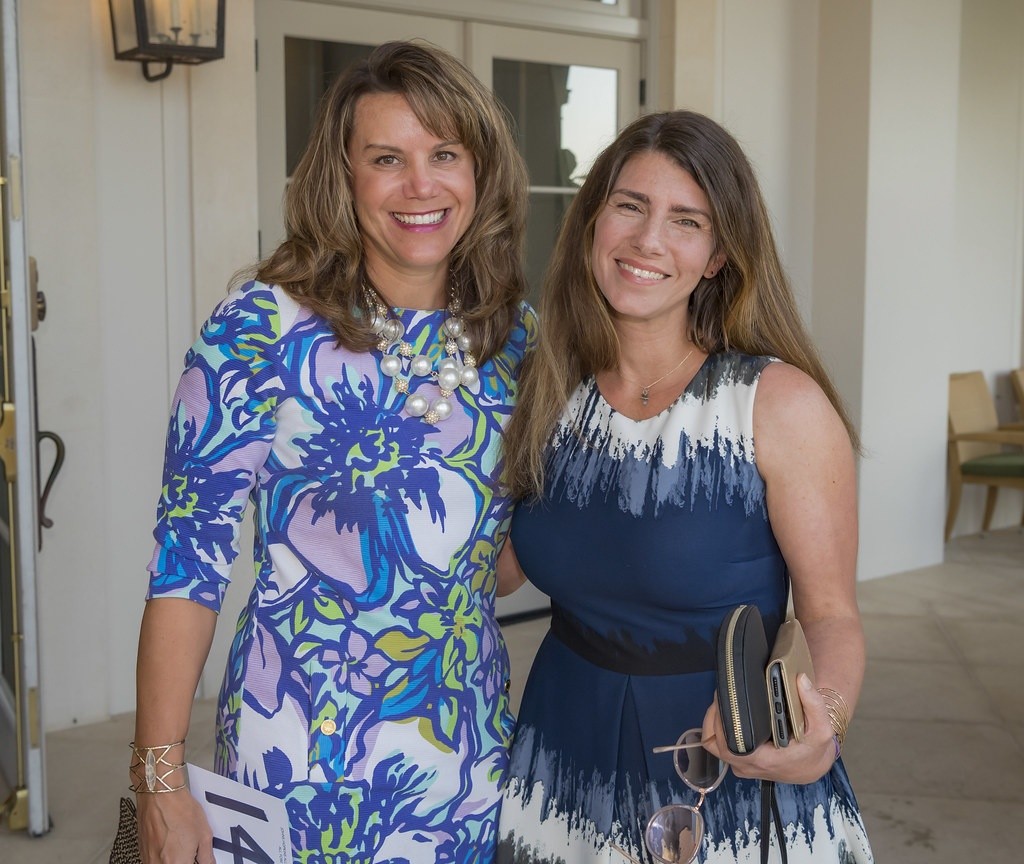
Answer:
494, 109, 874, 864
109, 41, 557, 864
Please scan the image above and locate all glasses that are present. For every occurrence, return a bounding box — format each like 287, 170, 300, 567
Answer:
608, 728, 730, 864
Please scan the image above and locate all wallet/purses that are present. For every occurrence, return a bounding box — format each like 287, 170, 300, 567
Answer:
717, 605, 771, 756
109, 797, 142, 864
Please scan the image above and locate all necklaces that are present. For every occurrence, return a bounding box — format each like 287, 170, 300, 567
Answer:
362, 270, 480, 428
611, 349, 696, 406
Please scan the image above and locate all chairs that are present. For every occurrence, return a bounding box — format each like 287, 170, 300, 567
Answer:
944, 372, 1024, 542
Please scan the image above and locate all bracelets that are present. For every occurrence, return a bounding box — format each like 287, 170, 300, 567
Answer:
815, 687, 855, 762
126, 739, 187, 797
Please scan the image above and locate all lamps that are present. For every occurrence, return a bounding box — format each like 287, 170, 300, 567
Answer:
109, 0, 226, 82
